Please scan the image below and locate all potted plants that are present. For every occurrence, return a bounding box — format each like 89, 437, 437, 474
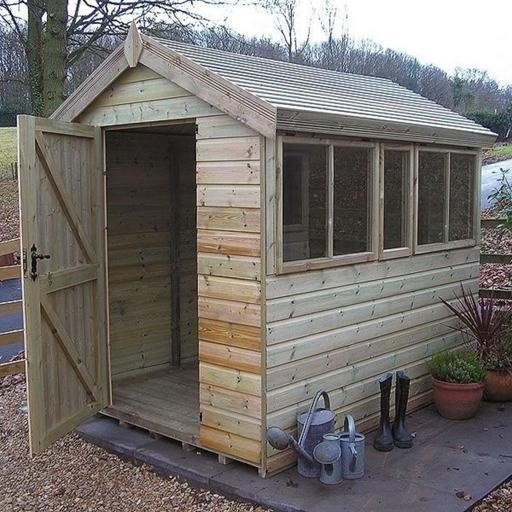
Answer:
439, 280, 511, 404
425, 339, 490, 427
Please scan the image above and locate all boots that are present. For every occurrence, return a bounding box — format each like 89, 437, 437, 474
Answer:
374, 373, 393, 451
393, 371, 413, 447
